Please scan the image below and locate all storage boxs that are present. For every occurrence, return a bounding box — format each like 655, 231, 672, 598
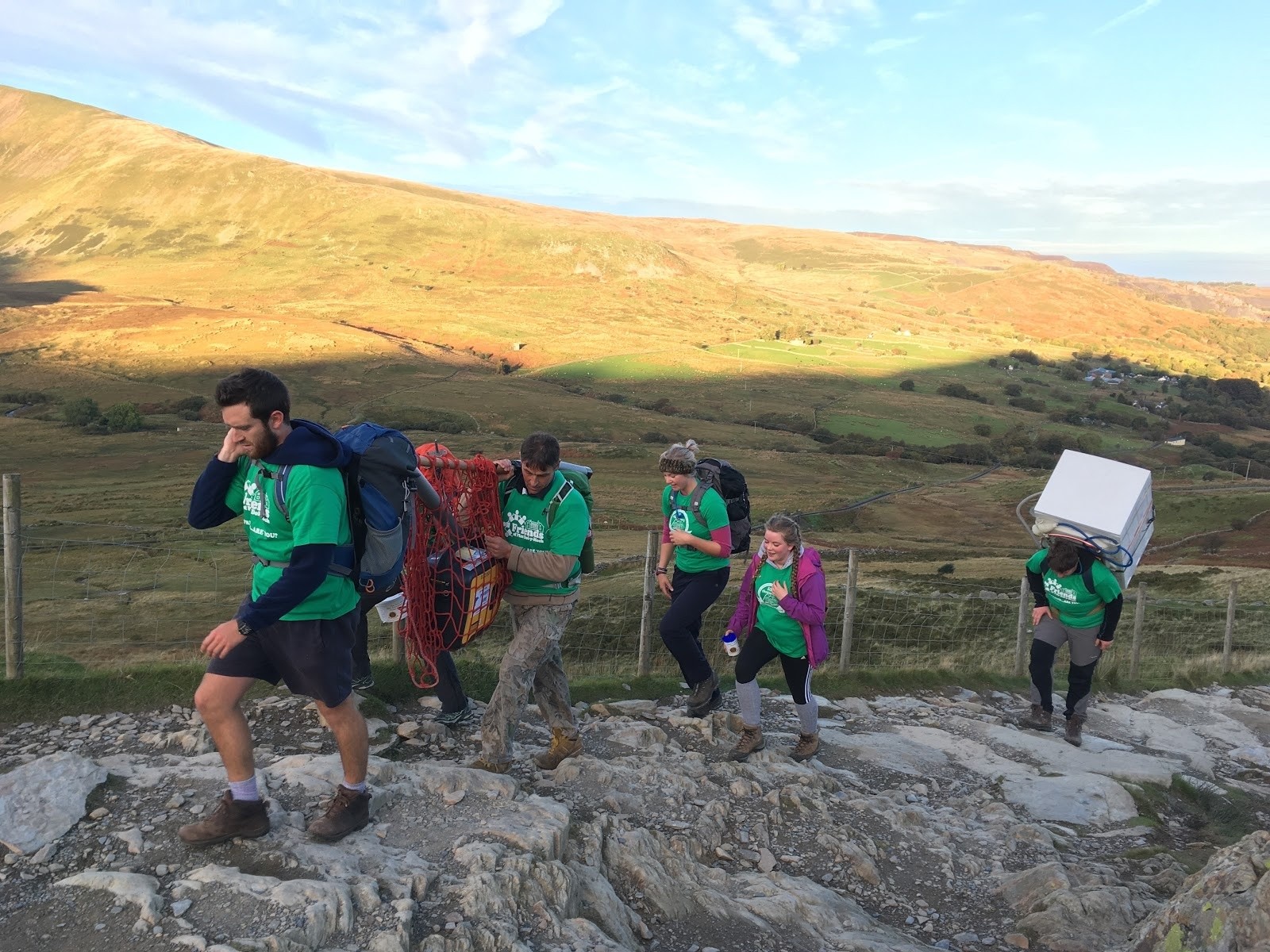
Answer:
1033, 449, 1155, 590
428, 543, 507, 649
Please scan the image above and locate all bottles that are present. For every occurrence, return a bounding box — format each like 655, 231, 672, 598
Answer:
723, 633, 741, 657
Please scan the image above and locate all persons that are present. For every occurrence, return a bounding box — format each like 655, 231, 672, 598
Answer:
657, 439, 731, 719
176, 370, 371, 844
405, 432, 588, 775
724, 515, 829, 762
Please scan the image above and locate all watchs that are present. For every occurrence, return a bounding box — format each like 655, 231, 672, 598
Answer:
656, 567, 667, 576
236, 615, 253, 637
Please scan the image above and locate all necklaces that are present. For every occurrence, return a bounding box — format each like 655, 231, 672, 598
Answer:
671, 489, 688, 522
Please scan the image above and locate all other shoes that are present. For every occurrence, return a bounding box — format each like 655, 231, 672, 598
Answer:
467, 758, 512, 774
1064, 715, 1085, 747
306, 784, 369, 844
532, 729, 584, 770
1019, 704, 1053, 732
177, 790, 270, 845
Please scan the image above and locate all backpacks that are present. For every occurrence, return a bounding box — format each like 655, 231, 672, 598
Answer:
502, 469, 596, 577
273, 417, 418, 608
668, 458, 751, 554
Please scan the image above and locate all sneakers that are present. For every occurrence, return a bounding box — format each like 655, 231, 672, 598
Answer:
686, 673, 720, 708
790, 727, 823, 764
688, 694, 723, 718
351, 673, 375, 690
432, 705, 474, 724
729, 723, 767, 760
